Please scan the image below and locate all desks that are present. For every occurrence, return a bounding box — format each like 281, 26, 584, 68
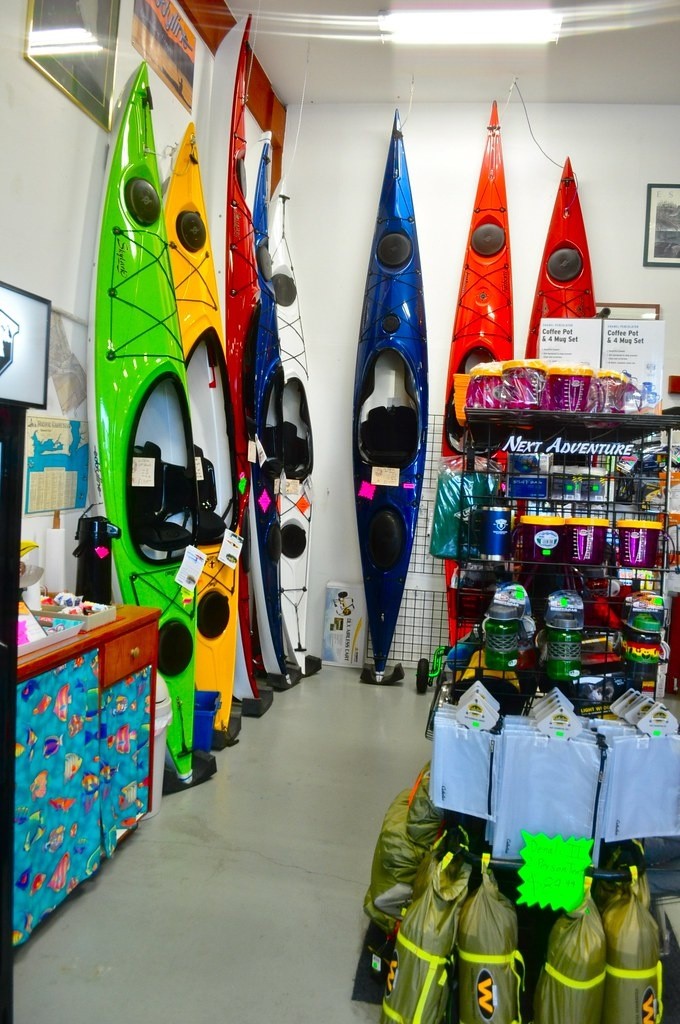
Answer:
11, 592, 162, 958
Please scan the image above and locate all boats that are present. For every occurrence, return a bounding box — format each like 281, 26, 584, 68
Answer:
442, 98, 595, 644
85, 14, 320, 795
350, 104, 429, 686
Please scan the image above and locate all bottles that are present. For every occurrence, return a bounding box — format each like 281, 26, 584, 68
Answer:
548, 621, 581, 680
485, 607, 517, 670
627, 618, 660, 679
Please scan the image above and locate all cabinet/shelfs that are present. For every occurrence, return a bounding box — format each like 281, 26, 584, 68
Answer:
423, 408, 680, 739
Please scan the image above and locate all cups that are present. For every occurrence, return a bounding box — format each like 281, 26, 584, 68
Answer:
466, 364, 503, 408
549, 368, 593, 413
564, 518, 609, 563
480, 507, 511, 561
598, 371, 628, 414
75, 516, 119, 603
617, 520, 663, 567
521, 515, 565, 562
503, 360, 547, 410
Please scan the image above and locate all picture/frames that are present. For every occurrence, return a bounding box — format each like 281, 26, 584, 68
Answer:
22, 0, 122, 133
643, 183, 680, 268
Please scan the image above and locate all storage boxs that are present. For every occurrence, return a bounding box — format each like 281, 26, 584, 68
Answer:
30, 602, 117, 631
